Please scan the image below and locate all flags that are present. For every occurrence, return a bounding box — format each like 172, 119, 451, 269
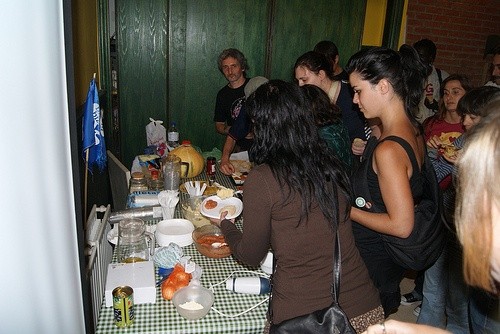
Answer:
83, 79, 106, 173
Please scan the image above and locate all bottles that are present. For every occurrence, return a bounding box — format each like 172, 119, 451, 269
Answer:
168, 122, 179, 148
129, 172, 148, 193
148, 171, 165, 191
169, 139, 204, 178
207, 157, 216, 174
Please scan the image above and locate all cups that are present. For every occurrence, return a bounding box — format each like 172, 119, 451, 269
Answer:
162, 154, 189, 190
112, 286, 135, 327
231, 172, 248, 184
162, 206, 175, 219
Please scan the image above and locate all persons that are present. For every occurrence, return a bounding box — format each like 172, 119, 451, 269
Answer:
215, 37, 500, 334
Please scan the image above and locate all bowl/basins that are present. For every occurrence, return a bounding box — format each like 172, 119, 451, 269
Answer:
173, 285, 215, 320
192, 225, 234, 258
260, 250, 274, 275
181, 197, 215, 228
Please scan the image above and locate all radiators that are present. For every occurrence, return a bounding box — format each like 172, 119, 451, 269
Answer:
82, 203, 115, 329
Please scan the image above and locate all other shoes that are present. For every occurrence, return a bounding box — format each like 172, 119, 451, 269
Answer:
400, 290, 423, 316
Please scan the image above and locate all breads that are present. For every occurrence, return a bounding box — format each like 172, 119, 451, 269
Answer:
217, 188, 234, 199
219, 206, 236, 217
229, 160, 252, 169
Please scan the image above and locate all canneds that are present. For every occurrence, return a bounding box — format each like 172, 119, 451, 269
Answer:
207, 157, 216, 175
112, 286, 135, 327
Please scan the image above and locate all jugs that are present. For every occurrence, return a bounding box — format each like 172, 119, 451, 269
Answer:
117, 217, 155, 262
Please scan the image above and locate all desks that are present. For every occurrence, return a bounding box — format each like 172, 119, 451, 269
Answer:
94, 160, 274, 333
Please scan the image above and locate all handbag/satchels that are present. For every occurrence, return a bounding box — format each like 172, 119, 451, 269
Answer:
356, 134, 447, 272
269, 307, 357, 334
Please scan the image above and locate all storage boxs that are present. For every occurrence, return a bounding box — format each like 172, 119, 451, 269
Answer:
105, 261, 156, 309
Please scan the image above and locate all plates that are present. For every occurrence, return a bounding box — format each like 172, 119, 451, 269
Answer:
156, 218, 195, 246
200, 195, 243, 219
107, 229, 150, 245
180, 181, 220, 195
